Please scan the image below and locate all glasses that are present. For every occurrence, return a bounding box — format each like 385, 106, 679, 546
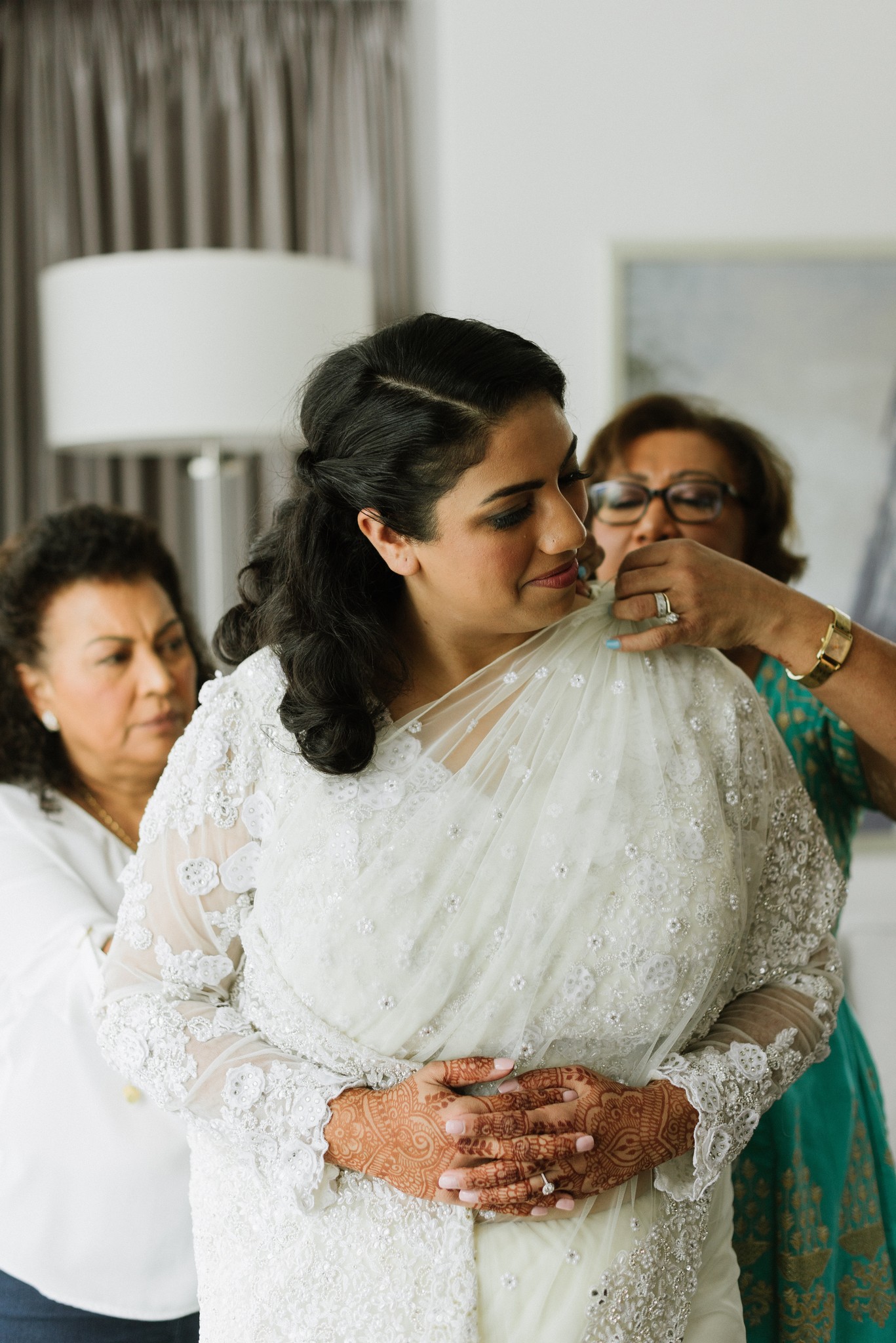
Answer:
586, 479, 752, 524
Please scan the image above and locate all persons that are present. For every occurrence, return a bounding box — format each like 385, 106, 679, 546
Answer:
576, 385, 896, 1343
90, 313, 846, 1343
1, 501, 212, 1343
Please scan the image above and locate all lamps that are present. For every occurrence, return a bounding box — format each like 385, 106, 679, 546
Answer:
39, 246, 375, 659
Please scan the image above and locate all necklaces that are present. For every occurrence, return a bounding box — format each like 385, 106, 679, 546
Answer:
74, 777, 138, 851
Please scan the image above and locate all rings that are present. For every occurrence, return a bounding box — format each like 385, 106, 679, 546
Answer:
654, 592, 667, 618
662, 592, 680, 625
540, 1171, 556, 1197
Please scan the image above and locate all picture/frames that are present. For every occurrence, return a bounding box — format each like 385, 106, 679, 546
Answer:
597, 232, 896, 859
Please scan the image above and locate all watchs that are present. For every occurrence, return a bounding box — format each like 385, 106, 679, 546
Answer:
785, 603, 853, 688
